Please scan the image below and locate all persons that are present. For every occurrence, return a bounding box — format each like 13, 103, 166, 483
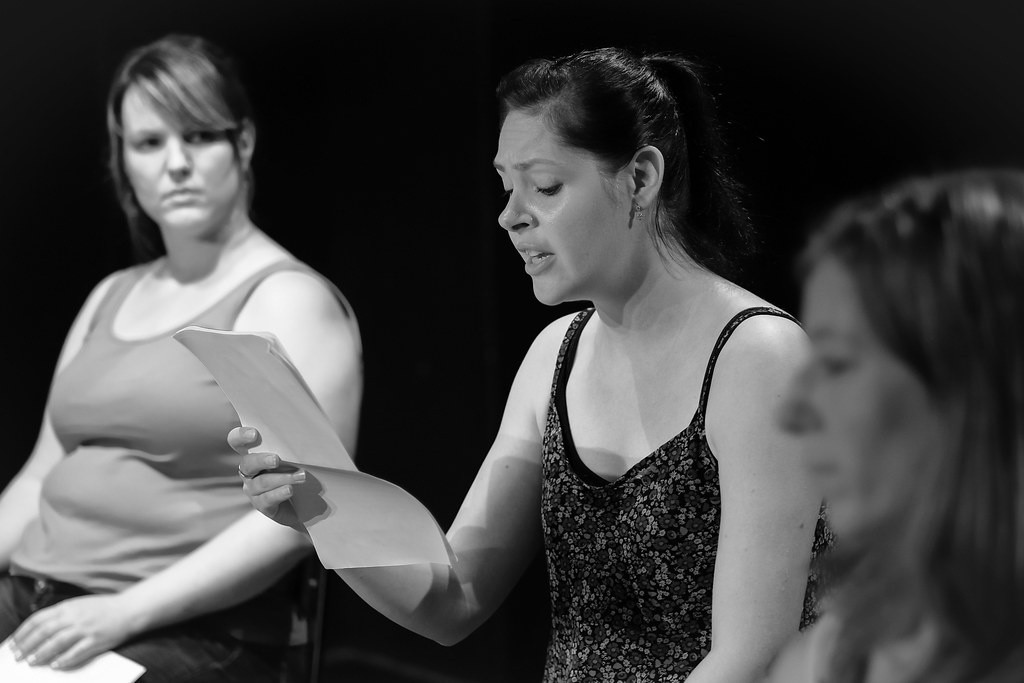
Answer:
2, 33, 366, 682
761, 180, 1024, 683
227, 48, 842, 681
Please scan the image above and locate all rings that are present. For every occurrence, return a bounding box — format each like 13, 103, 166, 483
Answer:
237, 464, 255, 479
